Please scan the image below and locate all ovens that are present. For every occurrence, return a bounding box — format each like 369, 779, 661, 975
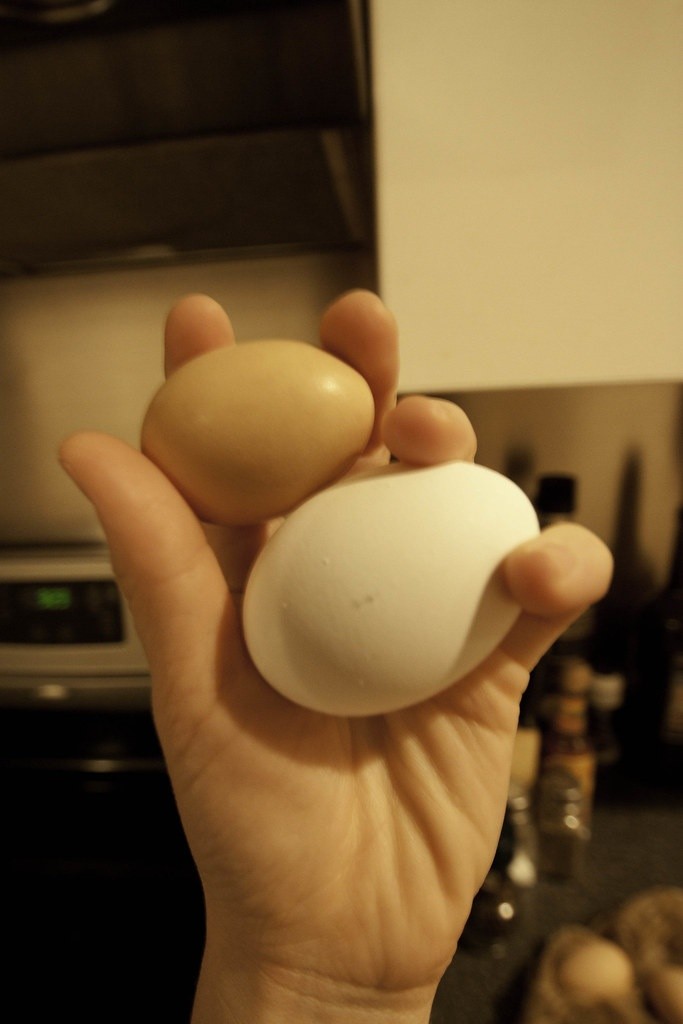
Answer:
0, 544, 150, 676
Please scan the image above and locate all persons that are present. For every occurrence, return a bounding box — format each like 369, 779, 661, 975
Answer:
58, 288, 613, 1024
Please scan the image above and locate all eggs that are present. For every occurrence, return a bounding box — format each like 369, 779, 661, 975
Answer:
244, 456, 542, 716
140, 339, 374, 525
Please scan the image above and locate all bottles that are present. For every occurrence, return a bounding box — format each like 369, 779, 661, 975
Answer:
539, 659, 595, 883
